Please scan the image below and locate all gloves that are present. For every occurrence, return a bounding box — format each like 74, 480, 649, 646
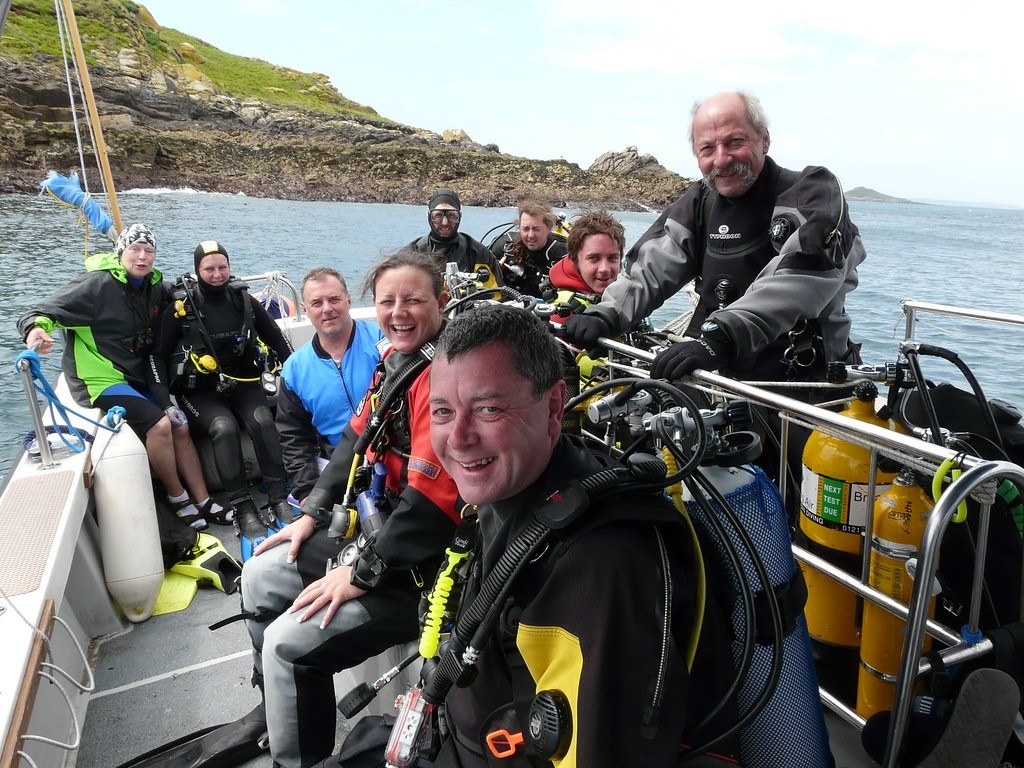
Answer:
561, 314, 610, 360
650, 338, 730, 383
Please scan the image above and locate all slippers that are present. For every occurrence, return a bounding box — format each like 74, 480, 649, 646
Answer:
201, 497, 234, 526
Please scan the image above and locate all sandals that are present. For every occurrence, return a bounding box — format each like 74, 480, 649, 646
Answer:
168, 498, 209, 532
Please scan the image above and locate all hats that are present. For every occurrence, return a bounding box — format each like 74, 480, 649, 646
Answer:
116, 224, 157, 265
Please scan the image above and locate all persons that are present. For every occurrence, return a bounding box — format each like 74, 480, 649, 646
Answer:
241, 251, 717, 768
560, 92, 867, 504
385, 187, 630, 338
16, 222, 302, 541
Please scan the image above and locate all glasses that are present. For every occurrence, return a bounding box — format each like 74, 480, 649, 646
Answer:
430, 209, 461, 224
